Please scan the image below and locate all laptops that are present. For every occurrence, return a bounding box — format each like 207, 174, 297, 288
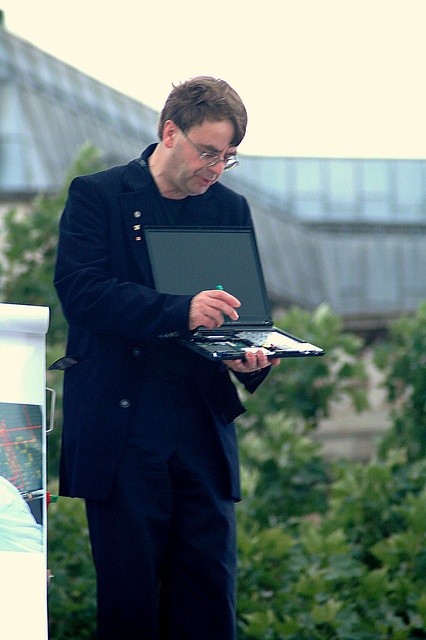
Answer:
142, 222, 326, 365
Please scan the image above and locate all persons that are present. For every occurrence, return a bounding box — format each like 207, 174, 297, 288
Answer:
48, 78, 281, 636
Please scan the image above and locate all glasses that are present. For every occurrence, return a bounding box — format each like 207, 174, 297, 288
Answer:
176, 125, 240, 170
